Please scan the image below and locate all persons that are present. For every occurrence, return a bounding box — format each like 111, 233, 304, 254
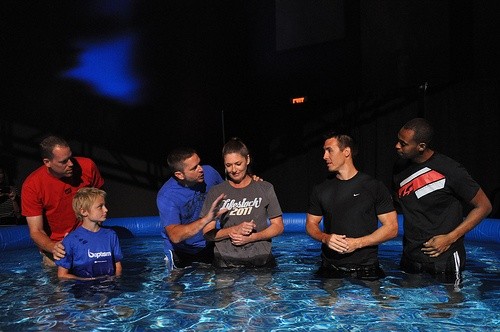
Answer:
156, 147, 263, 268
21, 135, 106, 267
199, 138, 284, 267
305, 135, 398, 275
55, 187, 123, 281
392, 118, 492, 274
